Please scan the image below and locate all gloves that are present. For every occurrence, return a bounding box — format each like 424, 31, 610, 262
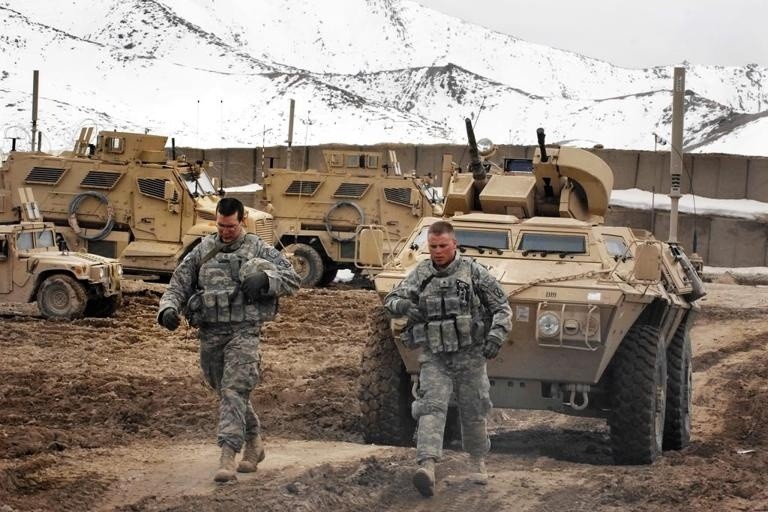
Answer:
481, 340, 499, 359
162, 308, 181, 330
240, 272, 267, 303
406, 305, 423, 322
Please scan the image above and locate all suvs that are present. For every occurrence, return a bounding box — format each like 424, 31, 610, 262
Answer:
0, 220, 124, 322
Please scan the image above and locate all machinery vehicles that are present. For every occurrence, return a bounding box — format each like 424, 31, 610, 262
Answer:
0, 68, 274, 283
261, 98, 442, 292
357, 66, 707, 466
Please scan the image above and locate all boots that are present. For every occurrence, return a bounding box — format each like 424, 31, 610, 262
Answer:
468, 454, 488, 484
213, 443, 237, 480
411, 458, 435, 495
237, 435, 265, 472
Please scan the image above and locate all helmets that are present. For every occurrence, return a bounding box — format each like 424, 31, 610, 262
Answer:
239, 258, 276, 283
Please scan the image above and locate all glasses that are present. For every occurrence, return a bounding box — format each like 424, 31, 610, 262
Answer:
216, 221, 241, 231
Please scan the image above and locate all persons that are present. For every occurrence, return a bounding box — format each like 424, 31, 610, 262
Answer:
384, 221, 514, 497
155, 198, 303, 482
